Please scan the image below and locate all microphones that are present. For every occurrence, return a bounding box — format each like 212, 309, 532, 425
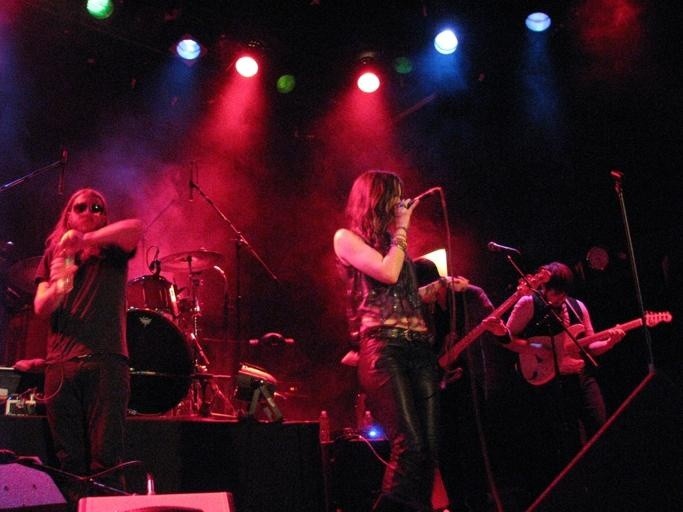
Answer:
58, 150, 68, 196
407, 187, 441, 209
488, 242, 521, 256
190, 161, 195, 201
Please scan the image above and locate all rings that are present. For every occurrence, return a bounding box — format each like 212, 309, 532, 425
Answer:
399, 203, 406, 208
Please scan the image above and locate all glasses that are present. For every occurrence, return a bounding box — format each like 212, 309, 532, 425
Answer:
73, 202, 104, 215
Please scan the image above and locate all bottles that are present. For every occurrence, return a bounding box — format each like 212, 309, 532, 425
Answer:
56, 243, 73, 296
320, 410, 329, 443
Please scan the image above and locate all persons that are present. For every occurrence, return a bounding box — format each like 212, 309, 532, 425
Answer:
27, 189, 143, 496
333, 169, 468, 512
500, 262, 627, 511
410, 259, 507, 512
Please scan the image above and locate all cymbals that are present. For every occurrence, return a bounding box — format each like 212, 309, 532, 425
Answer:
153, 250, 223, 273
9, 257, 44, 294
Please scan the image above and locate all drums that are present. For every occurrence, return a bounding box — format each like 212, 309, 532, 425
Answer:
126, 306, 197, 416
127, 274, 176, 322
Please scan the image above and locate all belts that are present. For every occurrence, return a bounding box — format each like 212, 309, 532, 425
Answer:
68, 351, 127, 363
357, 325, 434, 344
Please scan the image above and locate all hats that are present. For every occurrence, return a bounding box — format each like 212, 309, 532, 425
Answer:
413, 258, 441, 290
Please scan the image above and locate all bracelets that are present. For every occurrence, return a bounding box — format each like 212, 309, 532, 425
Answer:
391, 233, 405, 239
388, 238, 408, 252
394, 226, 407, 232
497, 328, 512, 345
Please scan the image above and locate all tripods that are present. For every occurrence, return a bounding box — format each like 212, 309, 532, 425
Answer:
170, 274, 236, 415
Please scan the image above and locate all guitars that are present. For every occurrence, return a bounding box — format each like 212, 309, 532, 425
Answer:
519, 309, 673, 387
430, 268, 553, 391
